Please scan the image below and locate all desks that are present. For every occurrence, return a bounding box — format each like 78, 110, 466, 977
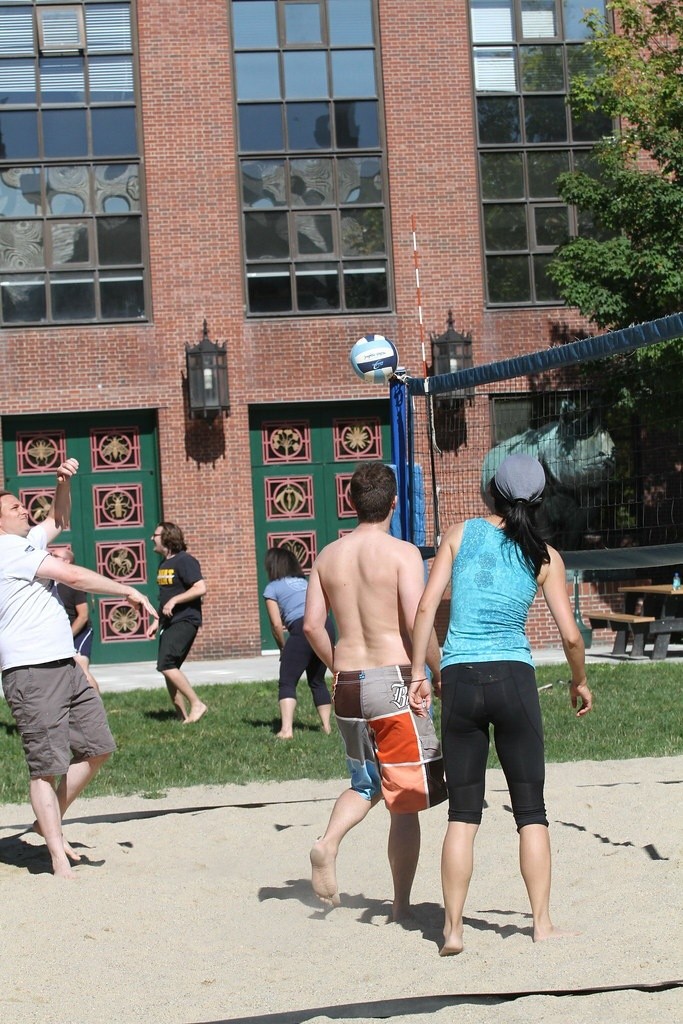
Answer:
611, 584, 683, 661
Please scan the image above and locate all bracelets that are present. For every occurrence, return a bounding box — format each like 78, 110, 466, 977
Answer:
411, 678, 428, 683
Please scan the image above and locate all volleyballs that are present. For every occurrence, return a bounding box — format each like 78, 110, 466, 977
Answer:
350, 334, 399, 384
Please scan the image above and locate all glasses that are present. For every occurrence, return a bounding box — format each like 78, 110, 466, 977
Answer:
154, 533, 163, 537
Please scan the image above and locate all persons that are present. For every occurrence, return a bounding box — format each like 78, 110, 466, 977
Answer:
48, 549, 102, 698
151, 522, 208, 724
303, 463, 441, 924
262, 548, 335, 739
0, 458, 160, 877
407, 454, 592, 956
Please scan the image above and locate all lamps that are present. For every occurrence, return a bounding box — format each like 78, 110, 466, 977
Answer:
429, 308, 477, 411
182, 318, 232, 420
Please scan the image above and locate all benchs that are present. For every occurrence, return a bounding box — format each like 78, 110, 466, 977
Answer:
582, 611, 669, 636
